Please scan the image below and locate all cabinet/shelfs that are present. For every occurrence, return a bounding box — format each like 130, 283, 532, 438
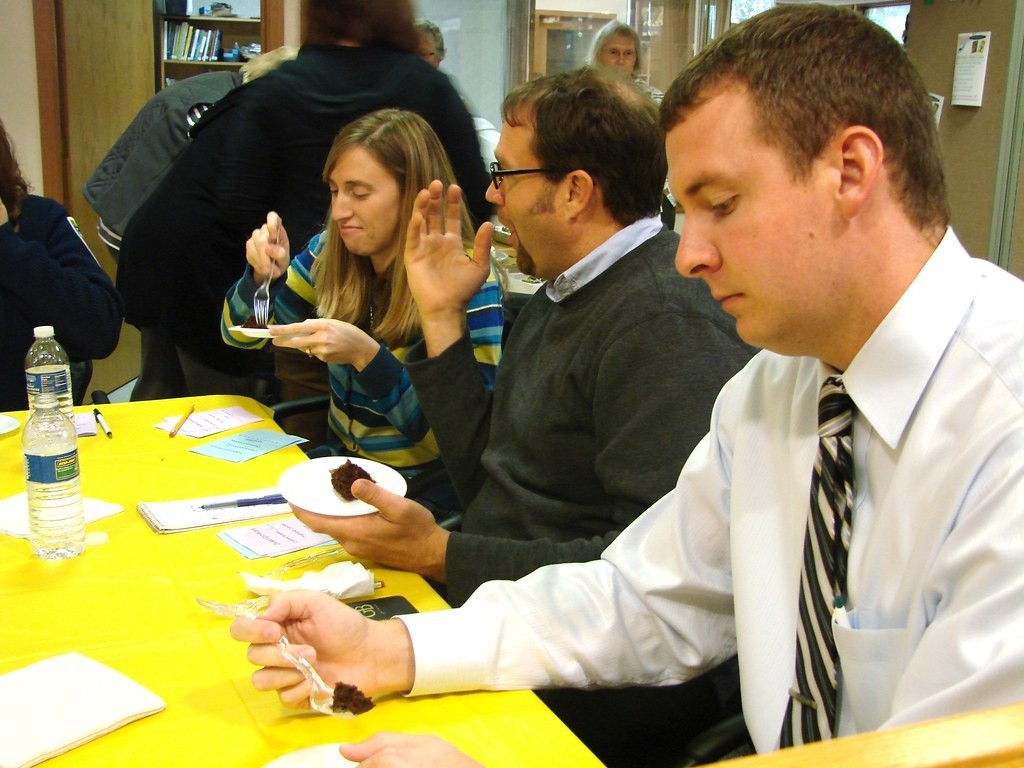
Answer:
156, 1, 268, 93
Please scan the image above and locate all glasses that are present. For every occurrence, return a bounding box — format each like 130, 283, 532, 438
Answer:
490, 162, 596, 190
419, 48, 435, 59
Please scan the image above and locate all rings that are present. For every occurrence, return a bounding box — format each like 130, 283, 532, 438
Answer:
306, 347, 313, 357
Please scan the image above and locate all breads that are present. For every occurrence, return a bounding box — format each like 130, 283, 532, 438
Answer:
331, 459, 377, 501
241, 314, 268, 329
330, 681, 375, 715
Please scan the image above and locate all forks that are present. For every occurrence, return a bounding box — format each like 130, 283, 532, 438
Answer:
195, 597, 270, 616
254, 218, 282, 323
278, 639, 352, 717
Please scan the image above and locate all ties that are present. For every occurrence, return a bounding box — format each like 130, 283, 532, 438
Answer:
774, 374, 858, 753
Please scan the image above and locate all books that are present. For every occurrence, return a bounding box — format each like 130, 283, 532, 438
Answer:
164, 21, 221, 61
136, 486, 292, 534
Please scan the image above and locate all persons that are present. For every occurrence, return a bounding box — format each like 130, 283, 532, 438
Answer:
0, 115, 123, 412
82, 44, 301, 402
587, 20, 663, 106
229, 3, 1024, 768
114, 60, 290, 394
288, 60, 761, 728
415, 17, 480, 118
217, 0, 494, 402
221, 108, 509, 480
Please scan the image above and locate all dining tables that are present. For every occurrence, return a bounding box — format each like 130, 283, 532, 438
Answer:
1, 392, 606, 768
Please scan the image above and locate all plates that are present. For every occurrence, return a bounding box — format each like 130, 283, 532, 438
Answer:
280, 457, 407, 516
228, 324, 280, 338
260, 743, 360, 768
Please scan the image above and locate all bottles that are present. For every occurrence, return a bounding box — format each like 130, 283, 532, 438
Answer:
21, 393, 87, 561
24, 325, 74, 423
